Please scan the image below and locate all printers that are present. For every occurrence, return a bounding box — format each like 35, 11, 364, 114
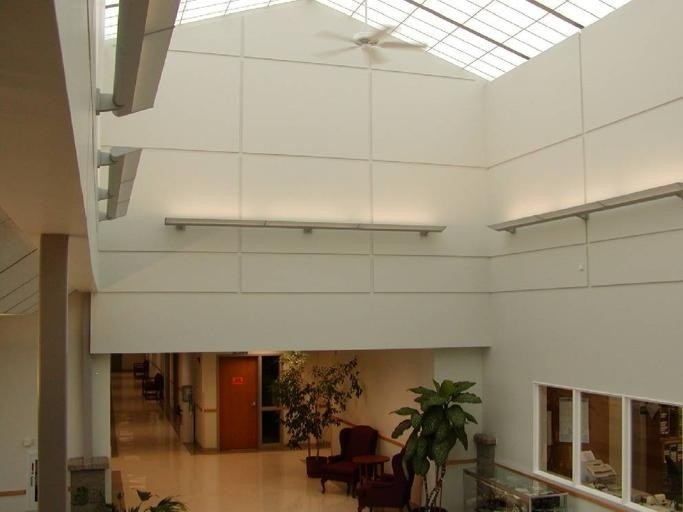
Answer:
582, 450, 616, 483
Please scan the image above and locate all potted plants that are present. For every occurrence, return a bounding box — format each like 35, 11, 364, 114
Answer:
386, 379, 482, 512
268, 350, 362, 477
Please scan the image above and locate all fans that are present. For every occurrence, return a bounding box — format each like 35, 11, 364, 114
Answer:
316, 1, 428, 67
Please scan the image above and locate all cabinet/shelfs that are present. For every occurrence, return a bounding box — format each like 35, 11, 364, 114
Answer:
644, 402, 683, 497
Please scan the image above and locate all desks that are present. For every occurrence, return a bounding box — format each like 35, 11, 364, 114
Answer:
353, 455, 389, 481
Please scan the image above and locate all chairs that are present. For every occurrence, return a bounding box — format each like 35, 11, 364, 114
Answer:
317, 425, 378, 499
132, 360, 148, 378
664, 454, 683, 496
355, 454, 411, 512
142, 373, 163, 401
